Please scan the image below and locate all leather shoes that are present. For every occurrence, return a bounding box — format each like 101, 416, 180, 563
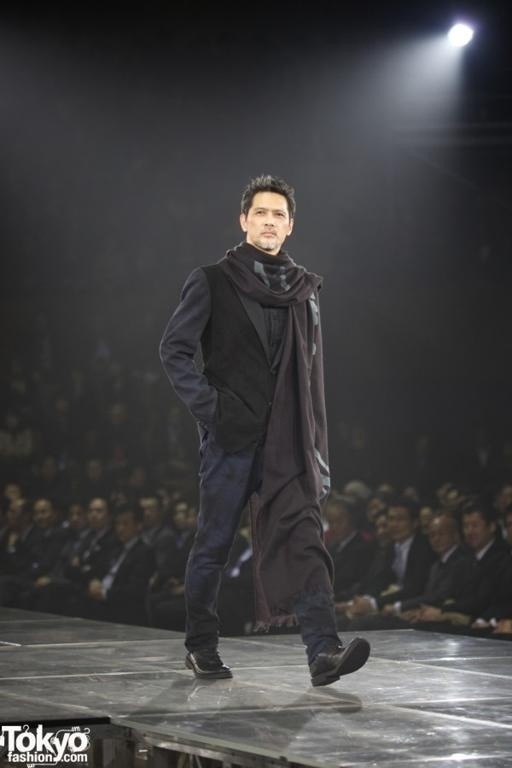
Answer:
308, 635, 371, 689
182, 649, 237, 681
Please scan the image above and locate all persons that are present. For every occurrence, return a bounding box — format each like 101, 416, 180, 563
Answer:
159, 173, 373, 687
1, 392, 509, 645
